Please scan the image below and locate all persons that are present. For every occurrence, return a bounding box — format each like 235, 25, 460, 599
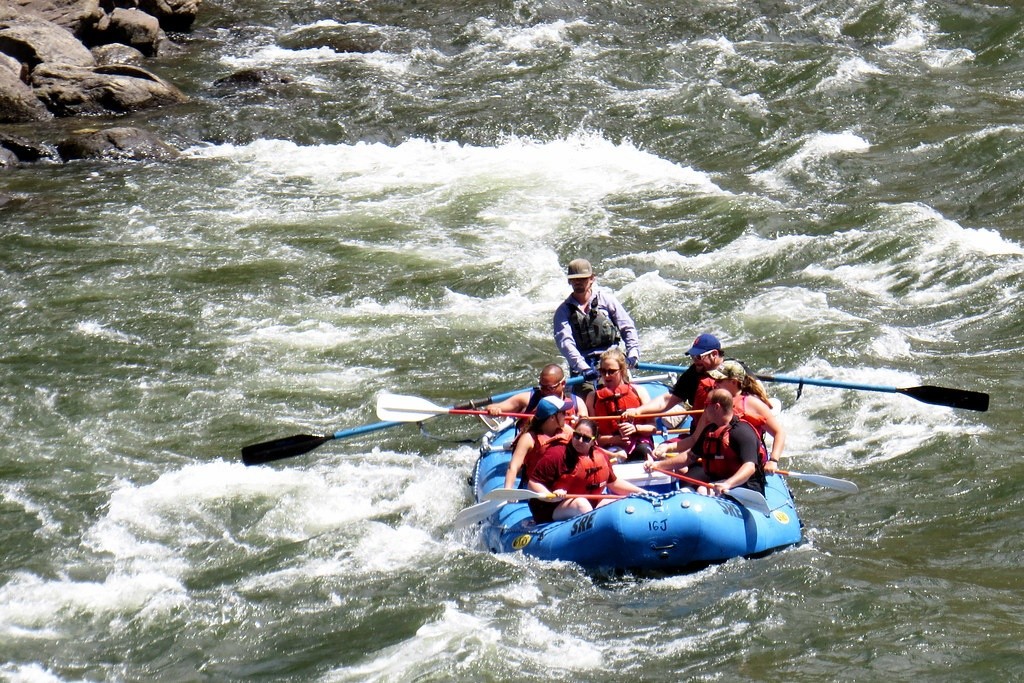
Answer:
642, 389, 767, 496
553, 259, 639, 401
586, 348, 659, 463
484, 363, 627, 503
621, 334, 784, 477
528, 418, 663, 524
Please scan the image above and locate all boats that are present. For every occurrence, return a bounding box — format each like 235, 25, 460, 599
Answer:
471, 385, 805, 578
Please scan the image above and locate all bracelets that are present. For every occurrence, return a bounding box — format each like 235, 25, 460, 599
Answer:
769, 458, 778, 463
634, 425, 638, 433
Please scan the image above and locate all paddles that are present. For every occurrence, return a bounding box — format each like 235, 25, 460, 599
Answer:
486, 488, 627, 504
455, 499, 500, 529
776, 469, 859, 497
374, 392, 782, 424
650, 465, 771, 514
635, 362, 991, 412
241, 369, 586, 466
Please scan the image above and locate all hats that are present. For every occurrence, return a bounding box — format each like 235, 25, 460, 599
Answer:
534, 395, 573, 420
706, 360, 746, 380
567, 258, 592, 279
685, 334, 720, 356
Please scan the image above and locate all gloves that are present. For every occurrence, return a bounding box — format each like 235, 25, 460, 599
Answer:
626, 356, 638, 369
581, 368, 599, 381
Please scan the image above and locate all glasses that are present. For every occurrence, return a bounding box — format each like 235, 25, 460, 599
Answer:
689, 350, 715, 360
598, 368, 620, 375
573, 432, 596, 443
539, 376, 564, 391
